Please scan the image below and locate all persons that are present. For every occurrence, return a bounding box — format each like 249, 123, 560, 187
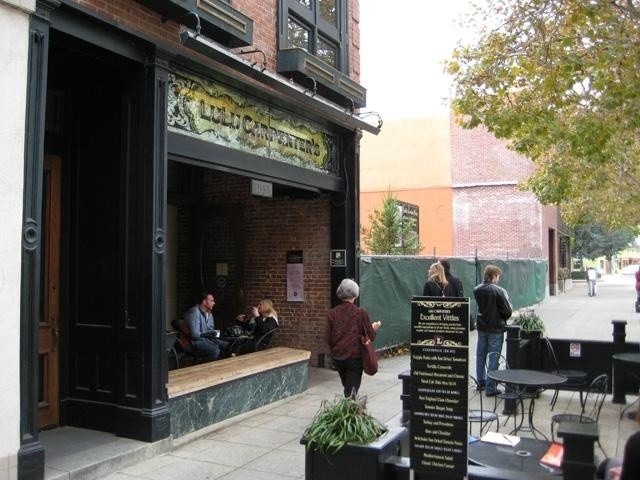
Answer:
607, 402, 640, 480
442, 261, 466, 332
182, 291, 221, 360
473, 265, 514, 396
586, 262, 600, 296
421, 260, 456, 298
237, 298, 279, 353
634, 265, 640, 313
323, 277, 382, 400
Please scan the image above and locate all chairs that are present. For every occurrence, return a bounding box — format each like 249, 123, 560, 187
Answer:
253, 329, 277, 352
482, 351, 529, 433
171, 319, 225, 365
544, 336, 588, 412
466, 375, 500, 438
550, 373, 612, 460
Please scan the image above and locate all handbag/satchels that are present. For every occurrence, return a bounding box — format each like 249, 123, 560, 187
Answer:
360, 335, 377, 376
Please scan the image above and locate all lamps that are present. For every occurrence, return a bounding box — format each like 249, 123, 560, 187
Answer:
236, 46, 267, 73
341, 98, 354, 114
159, 9, 202, 36
302, 76, 317, 96
358, 110, 382, 129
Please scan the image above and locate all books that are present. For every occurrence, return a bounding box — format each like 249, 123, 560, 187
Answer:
539, 443, 566, 468
480, 430, 521, 448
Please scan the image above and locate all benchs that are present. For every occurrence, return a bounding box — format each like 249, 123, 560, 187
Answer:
167, 346, 312, 439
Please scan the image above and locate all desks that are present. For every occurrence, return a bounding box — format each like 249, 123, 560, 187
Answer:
201, 328, 255, 358
467, 436, 598, 475
487, 368, 567, 443
613, 352, 640, 420
